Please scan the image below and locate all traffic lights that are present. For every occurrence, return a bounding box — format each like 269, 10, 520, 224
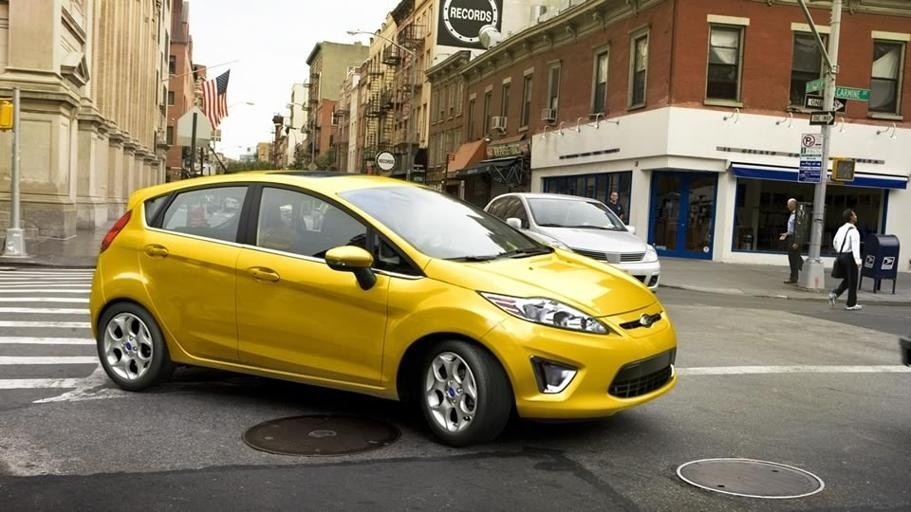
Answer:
830, 157, 855, 182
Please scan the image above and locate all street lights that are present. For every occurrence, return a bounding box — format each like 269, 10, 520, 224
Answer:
346, 27, 418, 185
212, 102, 256, 166
285, 102, 318, 172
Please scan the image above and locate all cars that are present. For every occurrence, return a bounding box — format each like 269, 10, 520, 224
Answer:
478, 191, 662, 297
86, 169, 681, 450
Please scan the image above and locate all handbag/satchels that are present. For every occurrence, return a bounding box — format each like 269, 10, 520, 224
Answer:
831, 256, 845, 278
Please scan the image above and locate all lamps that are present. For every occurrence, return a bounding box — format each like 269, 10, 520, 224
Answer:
723, 108, 897, 141
542, 113, 619, 137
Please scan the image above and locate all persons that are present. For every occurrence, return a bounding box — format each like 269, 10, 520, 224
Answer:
829, 208, 863, 310
605, 191, 624, 220
779, 198, 809, 284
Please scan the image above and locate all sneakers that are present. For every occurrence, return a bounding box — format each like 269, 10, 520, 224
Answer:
784, 278, 798, 284
844, 304, 862, 310
828, 292, 836, 308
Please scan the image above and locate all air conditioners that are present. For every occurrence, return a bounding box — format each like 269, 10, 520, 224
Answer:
541, 108, 556, 120
491, 116, 508, 132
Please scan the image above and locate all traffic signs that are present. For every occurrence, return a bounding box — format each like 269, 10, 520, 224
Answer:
835, 86, 873, 103
804, 78, 826, 93
806, 95, 847, 115
810, 111, 836, 126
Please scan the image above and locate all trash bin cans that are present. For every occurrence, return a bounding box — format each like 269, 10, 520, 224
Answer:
859, 234, 900, 294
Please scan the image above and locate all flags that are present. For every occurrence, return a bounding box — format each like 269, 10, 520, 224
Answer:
202, 69, 230, 131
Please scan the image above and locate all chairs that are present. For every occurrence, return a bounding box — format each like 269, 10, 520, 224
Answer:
320, 208, 360, 248
